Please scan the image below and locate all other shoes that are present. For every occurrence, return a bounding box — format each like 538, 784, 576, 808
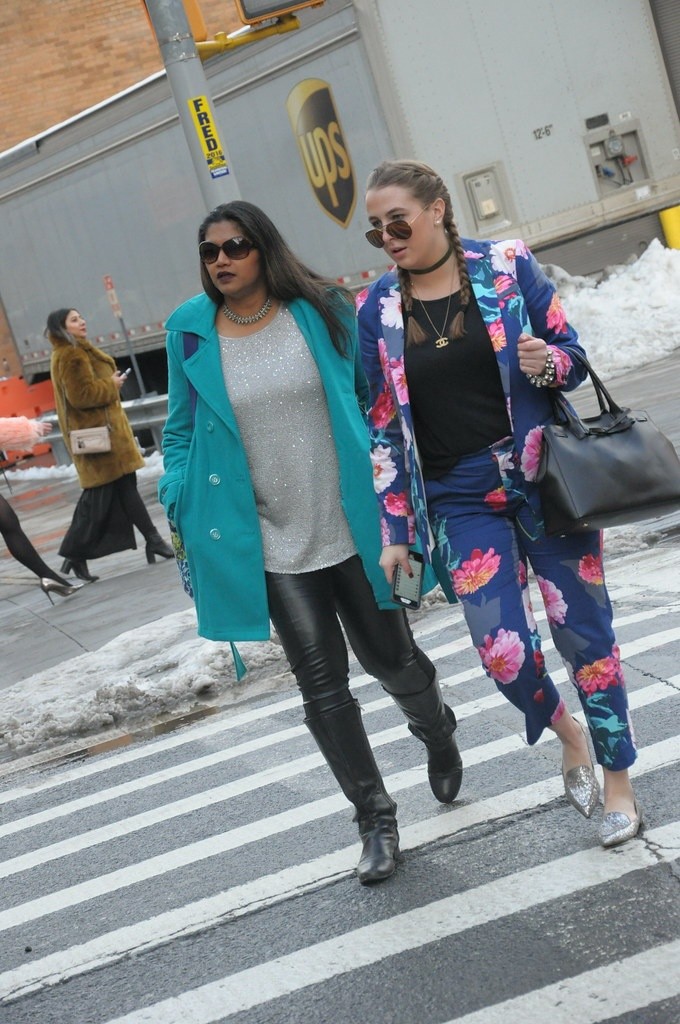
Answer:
554, 716, 601, 819
599, 799, 641, 846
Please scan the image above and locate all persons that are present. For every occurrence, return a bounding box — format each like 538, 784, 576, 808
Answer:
0, 414, 85, 604
354, 158, 643, 846
44, 309, 175, 581
158, 202, 463, 886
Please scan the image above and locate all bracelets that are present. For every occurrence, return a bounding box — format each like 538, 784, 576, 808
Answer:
525, 349, 555, 388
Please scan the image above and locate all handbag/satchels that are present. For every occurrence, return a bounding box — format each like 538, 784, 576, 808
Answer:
535, 347, 680, 540
70, 426, 112, 454
166, 503, 193, 599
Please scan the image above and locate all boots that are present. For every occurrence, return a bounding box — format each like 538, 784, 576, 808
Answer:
302, 698, 398, 885
382, 659, 464, 803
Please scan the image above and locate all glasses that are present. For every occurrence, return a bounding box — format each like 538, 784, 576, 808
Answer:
198, 236, 256, 264
363, 205, 429, 249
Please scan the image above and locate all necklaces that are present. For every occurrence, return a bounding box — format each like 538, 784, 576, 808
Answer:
400, 243, 458, 349
221, 297, 273, 323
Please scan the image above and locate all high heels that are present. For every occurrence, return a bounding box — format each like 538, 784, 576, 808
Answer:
59, 557, 100, 581
144, 529, 175, 564
40, 577, 85, 606
